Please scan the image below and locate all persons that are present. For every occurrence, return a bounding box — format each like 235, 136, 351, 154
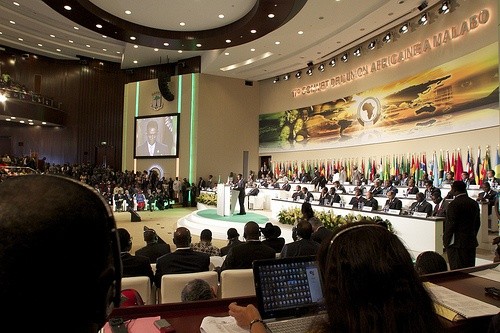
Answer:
137, 121, 169, 155
277, 108, 309, 149
492, 198, 500, 264
244, 161, 291, 192
0, 174, 123, 333
1, 69, 65, 110
476, 181, 497, 215
443, 180, 481, 270
300, 202, 315, 221
229, 172, 238, 185
291, 165, 455, 217
103, 216, 333, 332
228, 220, 443, 333
0, 152, 213, 212
416, 251, 448, 275
482, 169, 500, 190
460, 172, 476, 190
233, 174, 246, 215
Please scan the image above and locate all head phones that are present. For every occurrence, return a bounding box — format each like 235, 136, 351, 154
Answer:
50, 175, 123, 333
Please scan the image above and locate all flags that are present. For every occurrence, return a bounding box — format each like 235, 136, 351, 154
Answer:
273, 144, 500, 187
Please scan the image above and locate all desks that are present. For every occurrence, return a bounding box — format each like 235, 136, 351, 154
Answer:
200, 174, 500, 258
99, 262, 500, 333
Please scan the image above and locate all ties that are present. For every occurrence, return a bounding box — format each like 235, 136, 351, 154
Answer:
485, 192, 488, 197
418, 203, 420, 207
390, 200, 392, 204
489, 179, 491, 181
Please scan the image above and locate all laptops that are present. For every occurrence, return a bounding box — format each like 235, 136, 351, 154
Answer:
251, 253, 328, 333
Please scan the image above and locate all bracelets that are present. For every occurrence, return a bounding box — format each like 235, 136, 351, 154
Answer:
250, 320, 269, 333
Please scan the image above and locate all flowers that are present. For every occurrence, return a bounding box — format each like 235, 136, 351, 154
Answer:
277, 206, 394, 235
195, 193, 217, 206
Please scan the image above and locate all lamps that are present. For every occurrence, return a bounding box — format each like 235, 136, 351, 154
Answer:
273, 0, 449, 84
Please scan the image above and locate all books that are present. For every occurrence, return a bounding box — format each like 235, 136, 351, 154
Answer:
469, 263, 500, 282
422, 281, 500, 321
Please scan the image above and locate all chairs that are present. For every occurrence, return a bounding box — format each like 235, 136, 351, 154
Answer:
120, 268, 256, 304
